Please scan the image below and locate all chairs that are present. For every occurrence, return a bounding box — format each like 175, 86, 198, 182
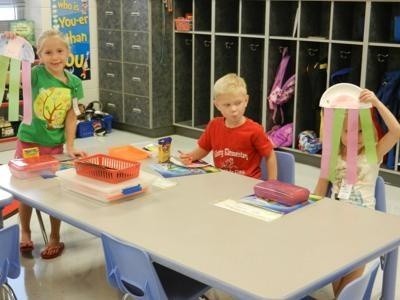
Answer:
99, 232, 212, 300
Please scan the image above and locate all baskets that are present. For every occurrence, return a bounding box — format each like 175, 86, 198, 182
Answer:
73, 154, 141, 184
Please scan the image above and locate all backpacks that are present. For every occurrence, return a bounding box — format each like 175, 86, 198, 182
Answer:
268, 47, 296, 124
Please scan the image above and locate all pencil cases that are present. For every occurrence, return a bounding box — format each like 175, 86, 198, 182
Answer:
254, 179, 310, 206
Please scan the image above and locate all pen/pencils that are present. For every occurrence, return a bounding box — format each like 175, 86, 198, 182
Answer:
177, 150, 183, 154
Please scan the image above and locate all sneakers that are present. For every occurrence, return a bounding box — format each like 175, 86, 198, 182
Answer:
76, 102, 108, 137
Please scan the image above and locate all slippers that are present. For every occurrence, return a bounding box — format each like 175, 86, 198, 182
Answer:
19, 240, 34, 253
41, 242, 64, 259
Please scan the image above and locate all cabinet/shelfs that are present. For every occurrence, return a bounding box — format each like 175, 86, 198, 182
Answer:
1, 60, 38, 143
96, 0, 175, 139
172, 0, 400, 186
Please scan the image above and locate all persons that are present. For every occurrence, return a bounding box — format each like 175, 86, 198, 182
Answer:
177, 71, 280, 184
1, 25, 86, 260
309, 85, 399, 300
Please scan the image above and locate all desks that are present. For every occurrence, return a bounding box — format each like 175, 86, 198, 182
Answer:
0, 142, 400, 299
